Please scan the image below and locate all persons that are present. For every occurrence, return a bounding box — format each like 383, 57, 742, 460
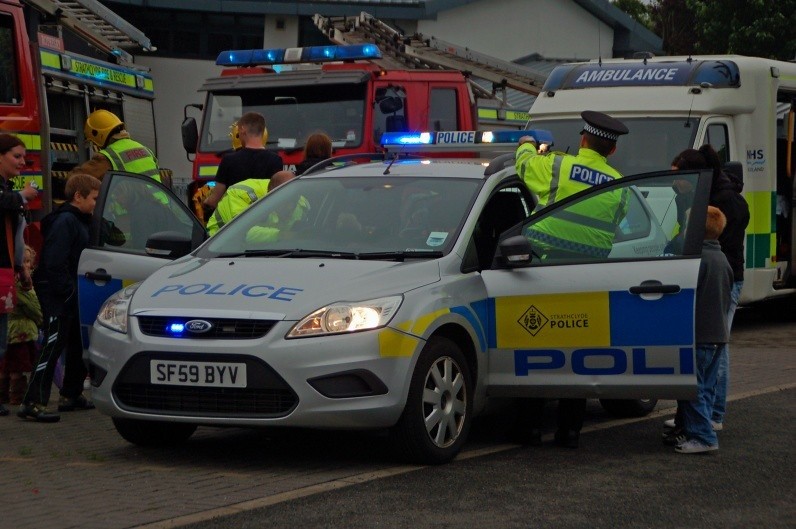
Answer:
67, 109, 170, 247
205, 170, 311, 243
0, 133, 39, 416
296, 132, 334, 175
506, 109, 631, 448
208, 111, 283, 211
664, 205, 734, 452
8, 245, 43, 406
338, 185, 456, 250
16, 173, 126, 423
665, 143, 750, 430
721, 161, 745, 192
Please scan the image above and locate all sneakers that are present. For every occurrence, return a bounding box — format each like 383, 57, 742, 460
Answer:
16, 402, 60, 422
58, 391, 95, 412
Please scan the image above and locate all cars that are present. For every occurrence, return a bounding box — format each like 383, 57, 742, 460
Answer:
77, 130, 718, 465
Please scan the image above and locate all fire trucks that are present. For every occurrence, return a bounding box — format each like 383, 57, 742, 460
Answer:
0, 0, 172, 219
180, 42, 483, 195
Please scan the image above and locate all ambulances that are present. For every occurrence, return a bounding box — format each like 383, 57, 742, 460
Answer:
521, 51, 795, 307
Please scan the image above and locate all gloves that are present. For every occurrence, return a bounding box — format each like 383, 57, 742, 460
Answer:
519, 135, 537, 148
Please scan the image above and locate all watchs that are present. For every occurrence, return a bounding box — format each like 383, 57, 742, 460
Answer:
523, 138, 535, 144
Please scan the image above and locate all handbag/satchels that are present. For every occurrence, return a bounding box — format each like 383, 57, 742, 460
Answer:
0, 268, 18, 314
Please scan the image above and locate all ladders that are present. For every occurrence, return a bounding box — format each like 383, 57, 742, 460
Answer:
311, 11, 547, 110
23, 0, 152, 63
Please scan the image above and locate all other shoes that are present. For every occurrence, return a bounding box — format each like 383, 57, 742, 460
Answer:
510, 420, 543, 448
554, 426, 580, 448
675, 439, 719, 453
662, 429, 688, 443
664, 417, 723, 431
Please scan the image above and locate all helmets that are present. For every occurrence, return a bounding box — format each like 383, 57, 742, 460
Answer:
84, 109, 125, 148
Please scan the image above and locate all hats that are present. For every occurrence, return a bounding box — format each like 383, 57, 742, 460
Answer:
579, 111, 629, 142
